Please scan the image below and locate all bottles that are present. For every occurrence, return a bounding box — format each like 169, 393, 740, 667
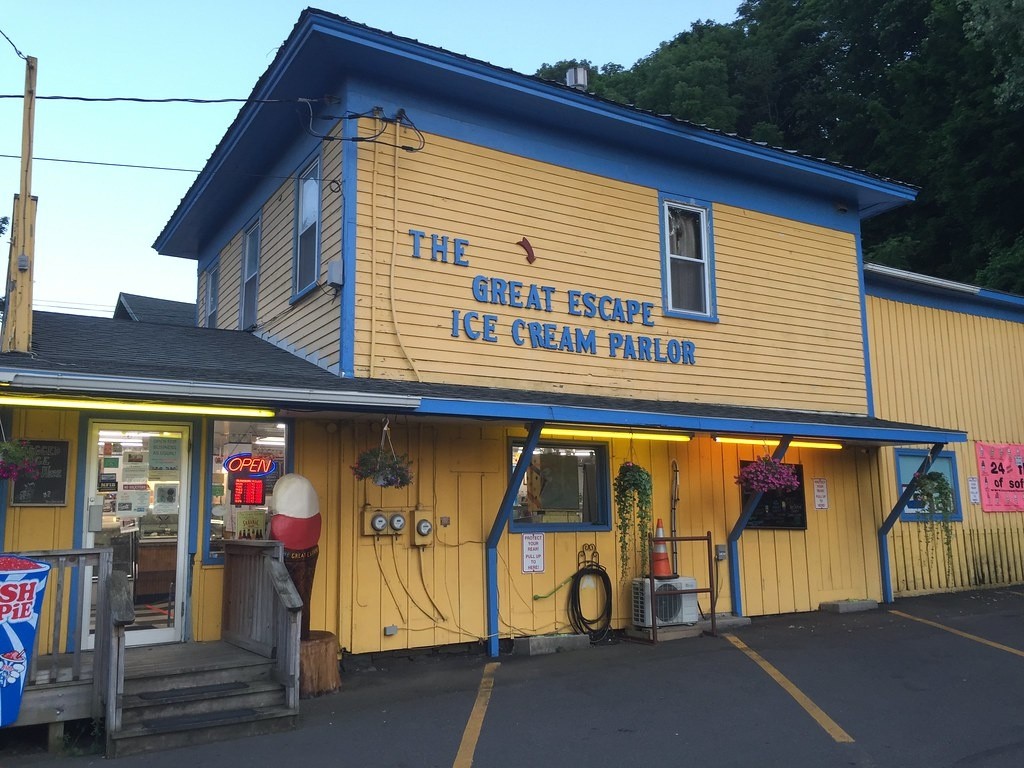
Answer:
238, 529, 263, 540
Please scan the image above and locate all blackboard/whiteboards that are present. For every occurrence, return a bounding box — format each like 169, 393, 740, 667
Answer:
739, 459, 808, 530
10, 438, 71, 506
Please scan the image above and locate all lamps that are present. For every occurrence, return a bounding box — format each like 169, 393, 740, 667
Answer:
523, 424, 696, 443
710, 435, 847, 450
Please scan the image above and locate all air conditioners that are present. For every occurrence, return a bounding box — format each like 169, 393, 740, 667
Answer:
631, 576, 699, 627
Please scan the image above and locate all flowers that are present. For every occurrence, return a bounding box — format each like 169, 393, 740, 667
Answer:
611, 460, 654, 603
912, 470, 957, 581
733, 436, 800, 495
349, 446, 416, 490
0, 436, 49, 484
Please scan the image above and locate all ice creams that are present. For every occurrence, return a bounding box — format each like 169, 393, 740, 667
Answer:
270, 472, 321, 640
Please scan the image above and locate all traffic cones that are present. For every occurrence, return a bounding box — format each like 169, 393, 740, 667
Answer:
644, 519, 680, 578
